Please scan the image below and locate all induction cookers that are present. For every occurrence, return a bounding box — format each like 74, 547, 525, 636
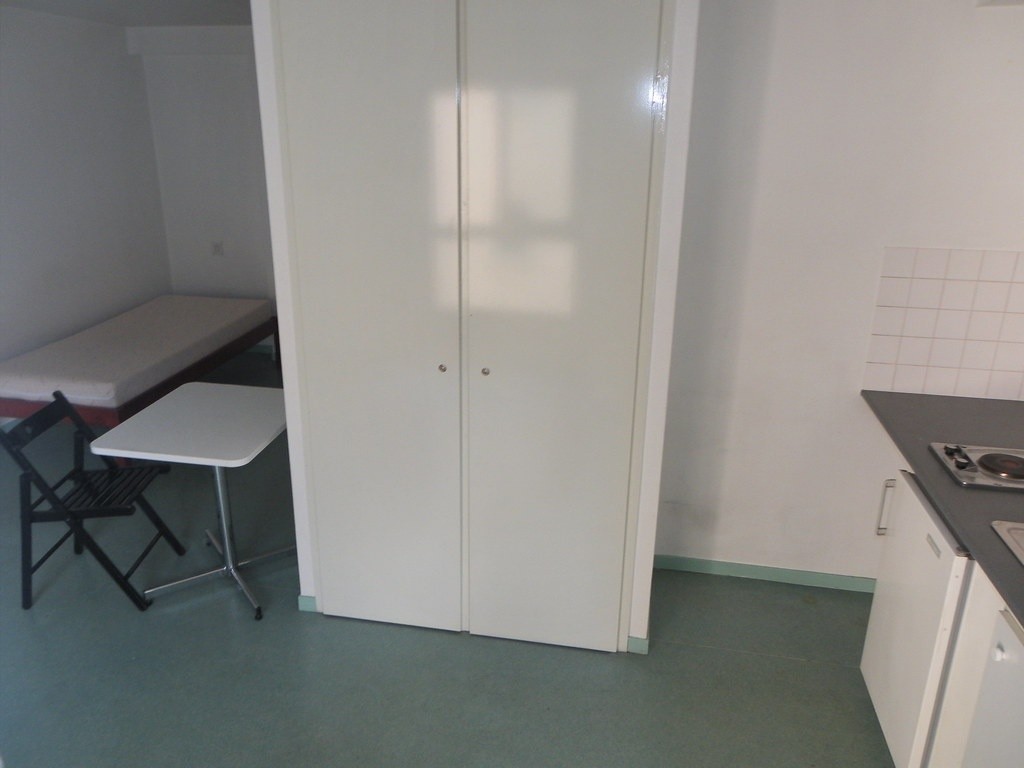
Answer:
929, 399, 1023, 585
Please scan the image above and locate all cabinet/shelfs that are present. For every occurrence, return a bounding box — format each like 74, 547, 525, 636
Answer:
251, 2, 702, 656
857, 469, 1024, 767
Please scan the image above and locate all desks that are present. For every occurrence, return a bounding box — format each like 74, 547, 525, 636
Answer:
89, 381, 296, 620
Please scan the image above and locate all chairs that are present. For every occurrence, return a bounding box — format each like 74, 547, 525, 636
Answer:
0, 390, 186, 612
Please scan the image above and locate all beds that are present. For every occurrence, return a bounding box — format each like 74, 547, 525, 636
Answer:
0, 292, 280, 475
